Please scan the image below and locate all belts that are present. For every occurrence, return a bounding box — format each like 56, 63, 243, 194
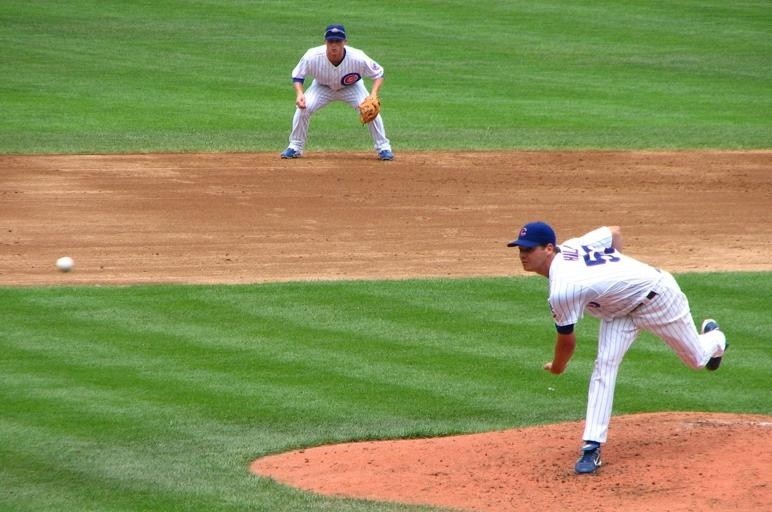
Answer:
638, 292, 656, 306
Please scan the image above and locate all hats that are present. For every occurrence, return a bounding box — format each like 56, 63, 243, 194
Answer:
325, 24, 345, 40
507, 222, 555, 247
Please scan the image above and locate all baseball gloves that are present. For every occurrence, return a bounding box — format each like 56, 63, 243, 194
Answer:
360, 96, 381, 126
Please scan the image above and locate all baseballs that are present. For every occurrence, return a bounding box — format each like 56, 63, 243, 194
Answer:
56, 256, 73, 273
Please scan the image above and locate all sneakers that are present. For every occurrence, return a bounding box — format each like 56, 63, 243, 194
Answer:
702, 319, 728, 370
575, 444, 601, 473
380, 150, 393, 160
281, 148, 301, 157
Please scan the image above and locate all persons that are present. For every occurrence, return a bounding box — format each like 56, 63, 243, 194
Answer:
280, 25, 395, 160
506, 221, 728, 472
